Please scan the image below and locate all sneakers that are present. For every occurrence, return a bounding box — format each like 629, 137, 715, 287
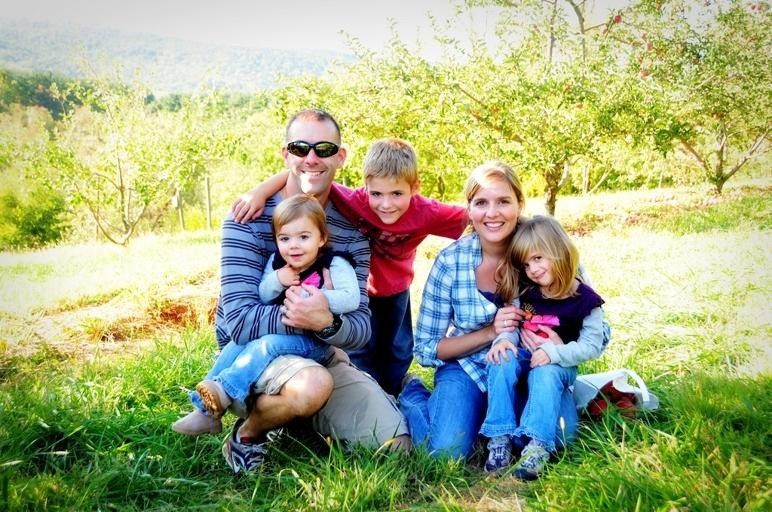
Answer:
512, 440, 550, 482
484, 435, 513, 474
222, 418, 277, 482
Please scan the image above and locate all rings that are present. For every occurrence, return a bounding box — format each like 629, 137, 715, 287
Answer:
279, 307, 288, 315
504, 320, 509, 329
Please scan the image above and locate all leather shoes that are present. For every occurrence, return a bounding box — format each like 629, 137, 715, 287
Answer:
196, 380, 232, 419
171, 410, 221, 435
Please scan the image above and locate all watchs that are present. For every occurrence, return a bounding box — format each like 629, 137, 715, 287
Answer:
318, 313, 342, 338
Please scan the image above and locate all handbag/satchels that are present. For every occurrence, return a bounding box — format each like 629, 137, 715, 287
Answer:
572, 369, 662, 413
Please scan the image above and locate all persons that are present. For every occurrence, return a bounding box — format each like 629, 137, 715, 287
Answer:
229, 133, 476, 396
478, 215, 607, 481
396, 163, 612, 468
168, 189, 361, 436
214, 107, 413, 475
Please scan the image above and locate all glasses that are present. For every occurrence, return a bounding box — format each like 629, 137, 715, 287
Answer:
287, 141, 339, 156
